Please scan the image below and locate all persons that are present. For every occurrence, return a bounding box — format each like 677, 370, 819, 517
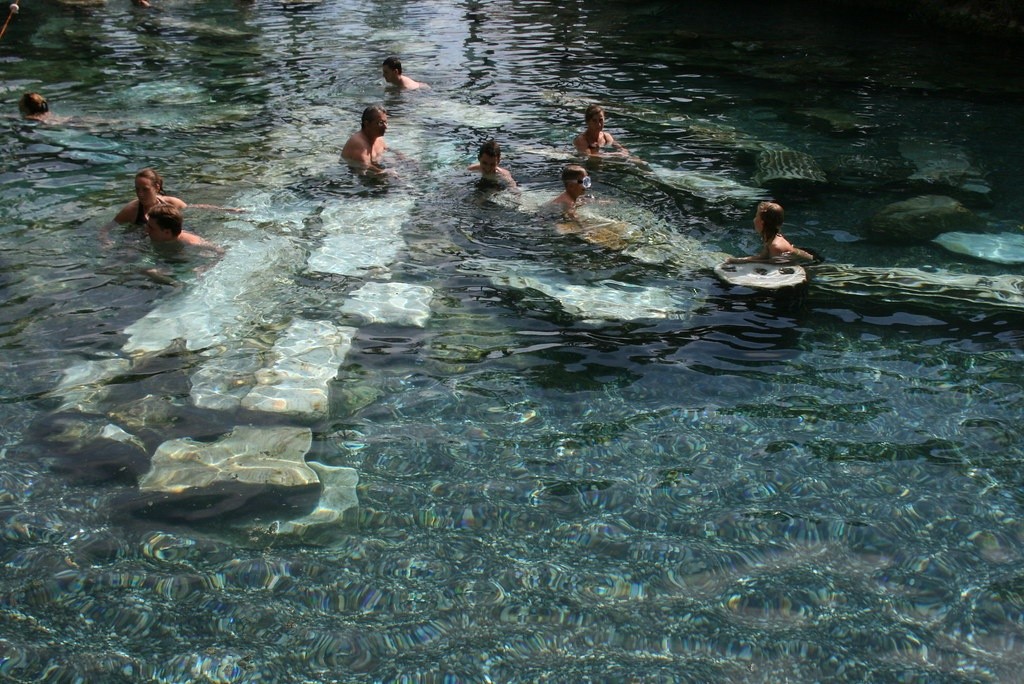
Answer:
572, 104, 629, 163
130, 0, 154, 8
382, 56, 431, 92
726, 201, 813, 267
98, 168, 248, 246
339, 105, 417, 178
17, 92, 100, 126
543, 165, 620, 230
103, 204, 227, 273
468, 139, 517, 191
